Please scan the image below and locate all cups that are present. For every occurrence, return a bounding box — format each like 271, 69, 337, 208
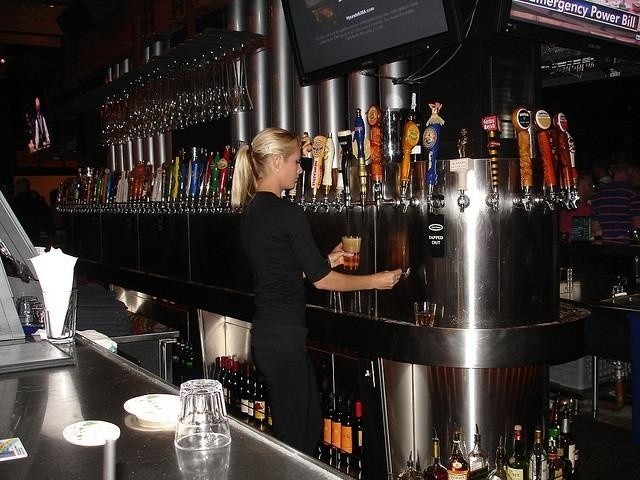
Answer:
173, 379, 232, 452
414, 300, 437, 328
341, 233, 362, 272
39, 288, 78, 344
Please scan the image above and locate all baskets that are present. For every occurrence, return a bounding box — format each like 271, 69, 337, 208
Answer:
549, 355, 612, 390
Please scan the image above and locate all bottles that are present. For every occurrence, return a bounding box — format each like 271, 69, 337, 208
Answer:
394, 398, 593, 479
211, 354, 275, 431
172, 339, 195, 384
317, 389, 363, 480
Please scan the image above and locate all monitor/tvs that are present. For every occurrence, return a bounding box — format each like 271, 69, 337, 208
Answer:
486, 0, 639, 64
278, 0, 464, 87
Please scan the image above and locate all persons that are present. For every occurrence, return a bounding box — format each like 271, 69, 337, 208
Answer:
230, 127, 402, 459
555, 156, 640, 246
33, 98, 50, 149
11, 177, 52, 251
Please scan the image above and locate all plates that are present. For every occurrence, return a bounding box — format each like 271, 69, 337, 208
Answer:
123, 393, 184, 424
121, 414, 179, 432
